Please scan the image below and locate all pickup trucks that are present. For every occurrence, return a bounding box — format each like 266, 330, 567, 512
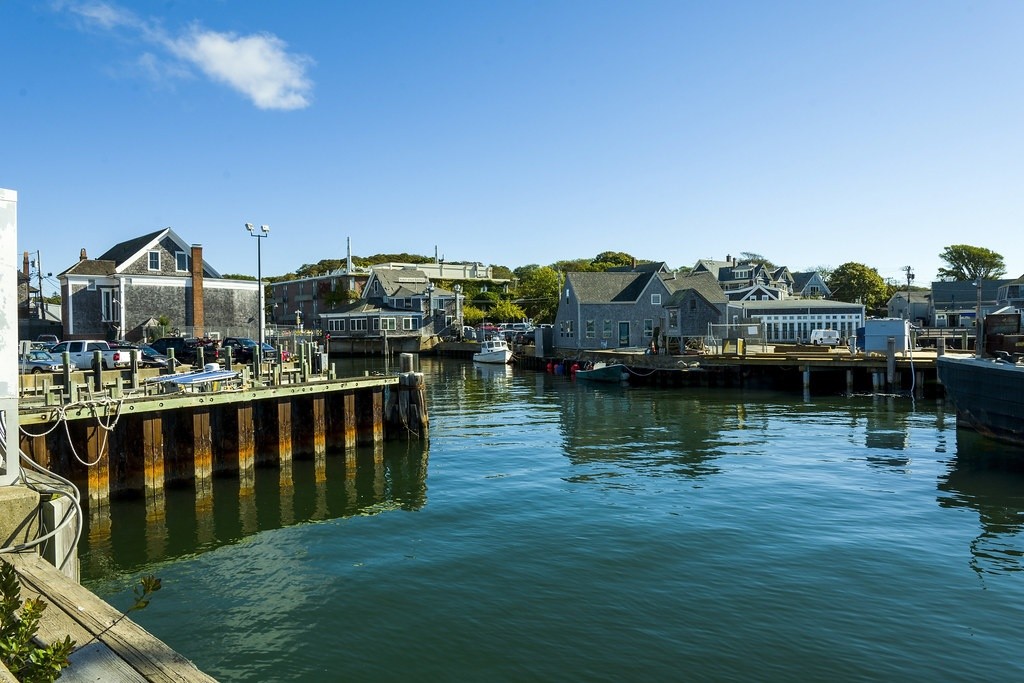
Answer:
149, 336, 236, 366
51, 338, 143, 370
221, 336, 265, 365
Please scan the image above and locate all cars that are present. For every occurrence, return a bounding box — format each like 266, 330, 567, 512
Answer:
462, 320, 553, 338
19, 348, 75, 373
254, 340, 291, 364
29, 333, 182, 369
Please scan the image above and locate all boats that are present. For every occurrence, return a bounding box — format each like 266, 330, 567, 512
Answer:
471, 335, 513, 365
575, 362, 625, 385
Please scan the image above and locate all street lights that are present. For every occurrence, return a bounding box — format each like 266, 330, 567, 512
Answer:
246, 222, 271, 347
